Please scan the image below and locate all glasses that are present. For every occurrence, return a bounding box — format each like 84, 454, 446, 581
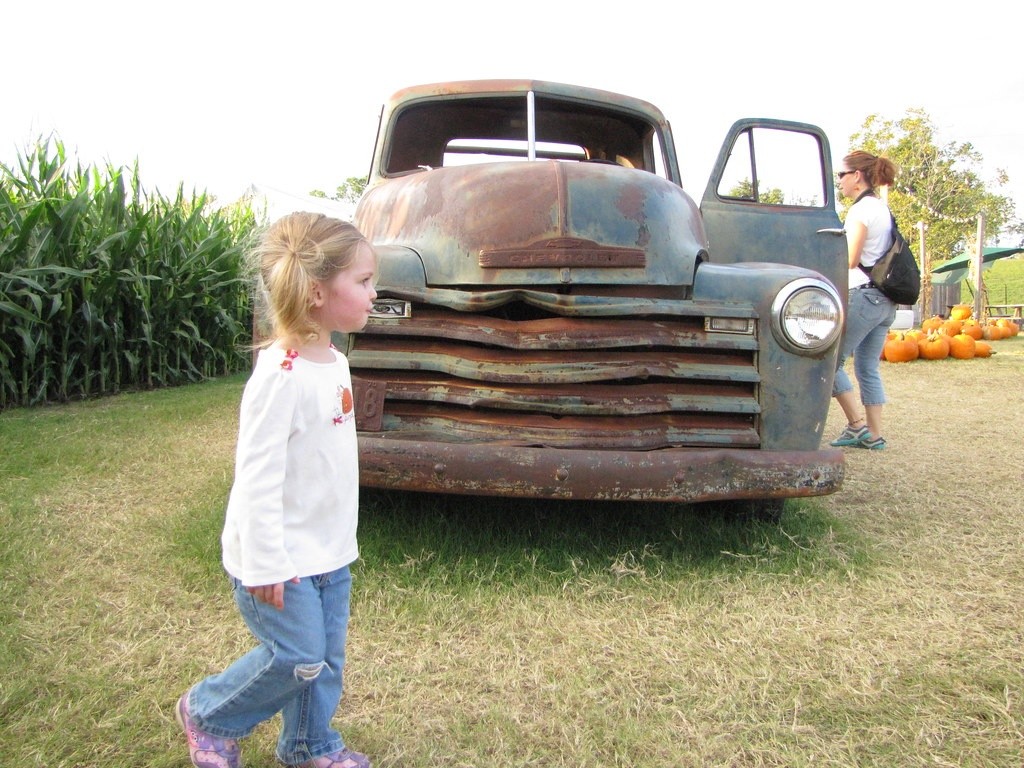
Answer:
837, 170, 855, 180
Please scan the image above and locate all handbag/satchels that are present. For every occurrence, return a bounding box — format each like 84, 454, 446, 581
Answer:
857, 205, 921, 305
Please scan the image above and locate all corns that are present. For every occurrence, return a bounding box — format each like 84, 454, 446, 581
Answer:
66, 277, 174, 320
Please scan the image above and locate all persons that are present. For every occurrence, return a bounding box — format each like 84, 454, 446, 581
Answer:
174, 210, 377, 768
829, 151, 899, 450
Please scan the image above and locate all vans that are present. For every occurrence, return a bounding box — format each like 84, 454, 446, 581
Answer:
252, 78, 852, 521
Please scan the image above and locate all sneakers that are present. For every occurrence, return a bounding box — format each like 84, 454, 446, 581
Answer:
829, 423, 870, 445
276, 744, 370, 767
175, 687, 241, 768
851, 437, 886, 449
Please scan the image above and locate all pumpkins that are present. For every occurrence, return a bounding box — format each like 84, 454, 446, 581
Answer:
879, 302, 1020, 363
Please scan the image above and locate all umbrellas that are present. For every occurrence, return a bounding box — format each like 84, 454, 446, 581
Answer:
931, 246, 1024, 317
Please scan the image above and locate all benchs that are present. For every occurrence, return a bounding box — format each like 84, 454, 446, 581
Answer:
988, 316, 1022, 323
989, 314, 1013, 317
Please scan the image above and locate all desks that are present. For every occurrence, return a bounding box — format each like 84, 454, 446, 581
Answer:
984, 304, 1024, 330
